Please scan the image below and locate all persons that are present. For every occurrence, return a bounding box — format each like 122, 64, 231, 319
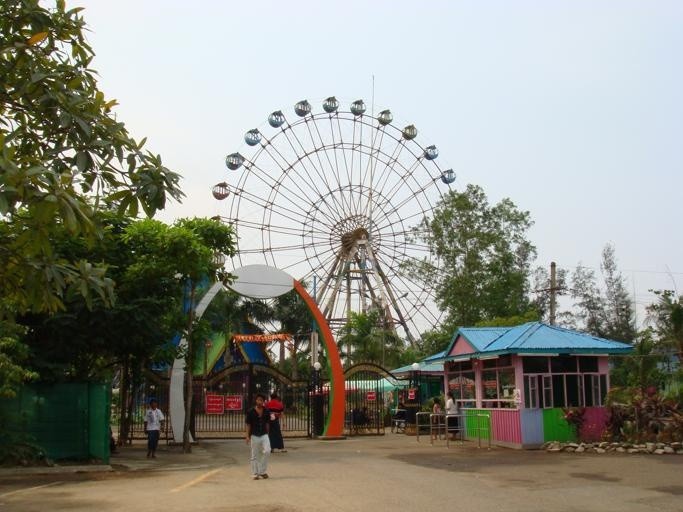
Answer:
445, 390, 460, 441
266, 394, 289, 454
244, 393, 279, 481
142, 398, 166, 458
431, 397, 442, 440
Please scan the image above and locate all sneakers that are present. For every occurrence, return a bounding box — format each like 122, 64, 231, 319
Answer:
260, 473, 268, 479
252, 475, 258, 480
